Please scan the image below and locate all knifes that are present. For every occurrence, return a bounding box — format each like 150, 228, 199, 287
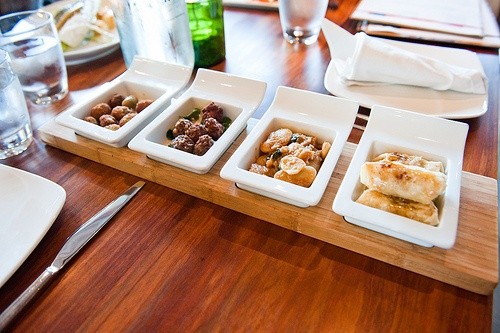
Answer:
0, 181, 145, 330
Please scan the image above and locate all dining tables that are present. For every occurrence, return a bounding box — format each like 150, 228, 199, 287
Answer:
0, 0, 500, 333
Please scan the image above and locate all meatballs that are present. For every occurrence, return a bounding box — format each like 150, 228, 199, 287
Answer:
84, 93, 153, 130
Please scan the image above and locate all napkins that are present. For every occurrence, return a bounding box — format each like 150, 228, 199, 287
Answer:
319, 19, 488, 93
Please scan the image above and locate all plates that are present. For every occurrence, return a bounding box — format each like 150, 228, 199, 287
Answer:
0, 163, 66, 289
329, 105, 471, 251
126, 67, 269, 175
52, 54, 194, 148
324, 17, 489, 119
12, 0, 128, 67
218, 86, 359, 208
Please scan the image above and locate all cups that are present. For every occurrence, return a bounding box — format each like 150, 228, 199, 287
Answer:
0, 48, 33, 160
279, 0, 334, 45
0, 11, 70, 103
111, 0, 225, 70
